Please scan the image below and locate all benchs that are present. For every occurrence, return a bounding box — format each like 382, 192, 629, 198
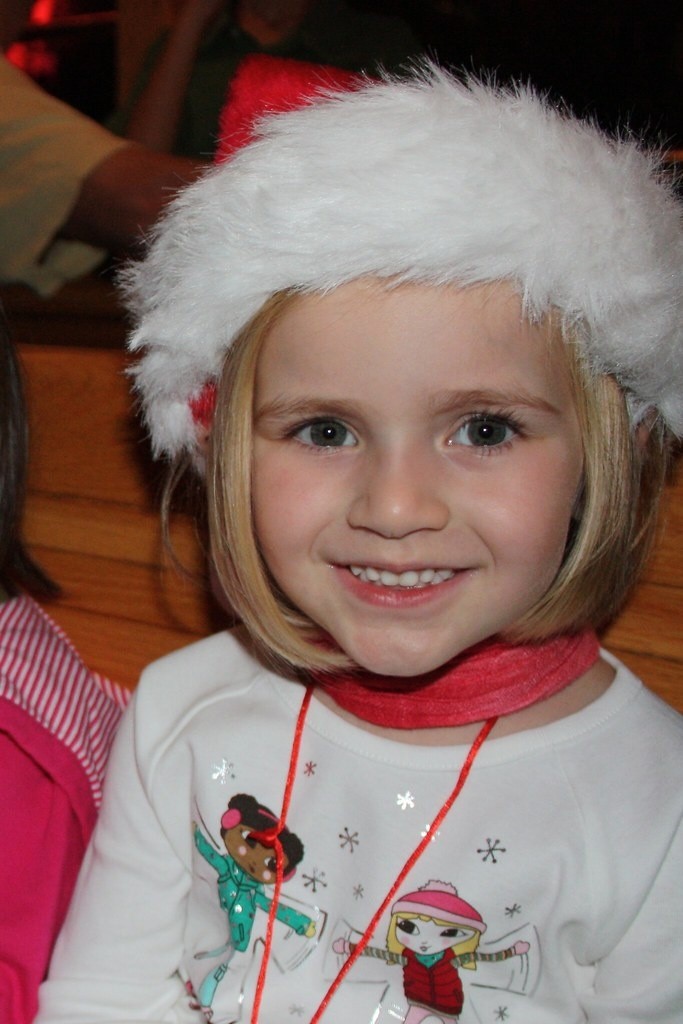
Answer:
17, 344, 683, 708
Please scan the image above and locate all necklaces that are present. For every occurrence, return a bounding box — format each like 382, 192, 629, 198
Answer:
249, 681, 495, 1024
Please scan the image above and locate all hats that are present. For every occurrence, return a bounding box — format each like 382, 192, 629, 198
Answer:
118, 58, 683, 459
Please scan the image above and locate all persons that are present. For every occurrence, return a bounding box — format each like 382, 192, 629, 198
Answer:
1, 0, 401, 299
0, 321, 131, 1024
35, 54, 683, 1024
